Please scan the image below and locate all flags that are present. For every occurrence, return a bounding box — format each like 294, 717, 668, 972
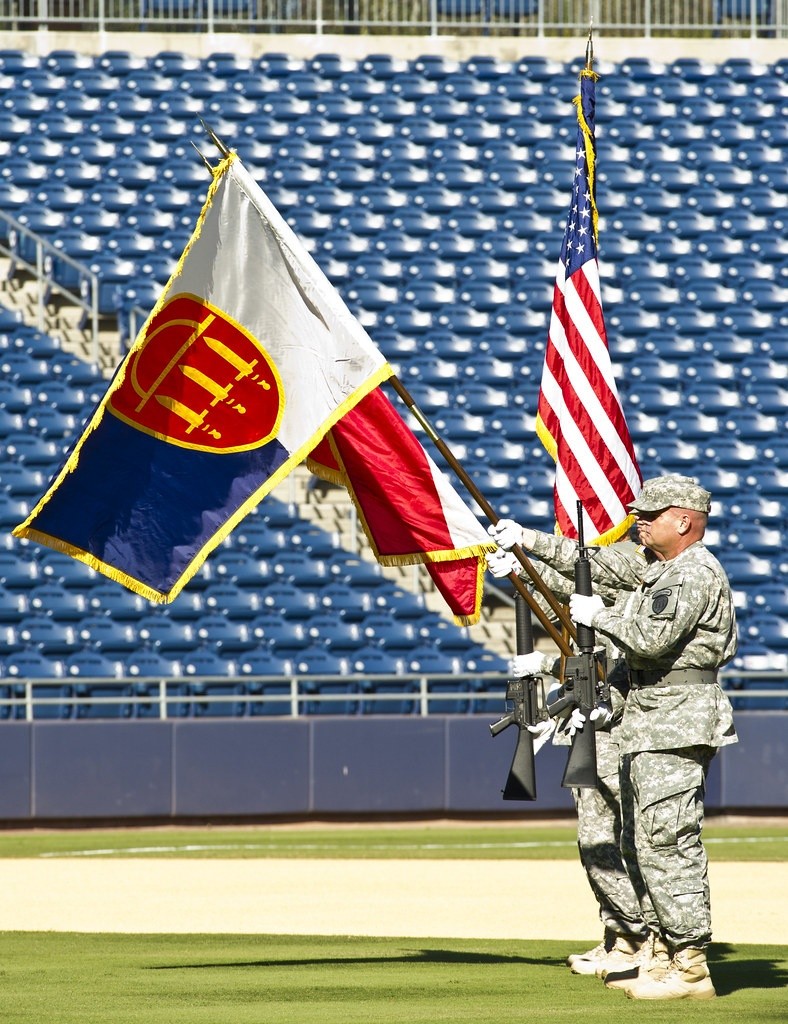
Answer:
305, 386, 497, 627
535, 69, 644, 547
11, 148, 396, 607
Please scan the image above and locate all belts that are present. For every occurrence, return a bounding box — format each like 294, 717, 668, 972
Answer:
624, 668, 719, 687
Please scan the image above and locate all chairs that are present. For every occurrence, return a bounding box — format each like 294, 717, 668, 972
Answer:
0, 50, 787, 722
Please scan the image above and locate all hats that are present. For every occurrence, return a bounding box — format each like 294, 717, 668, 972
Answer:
626, 476, 713, 514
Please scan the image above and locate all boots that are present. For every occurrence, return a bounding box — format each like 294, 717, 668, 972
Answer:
566, 934, 717, 1001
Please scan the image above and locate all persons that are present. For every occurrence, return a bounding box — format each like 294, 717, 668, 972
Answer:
484, 473, 740, 1001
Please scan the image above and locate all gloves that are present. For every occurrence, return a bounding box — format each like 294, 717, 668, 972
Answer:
546, 683, 564, 706
487, 518, 526, 549
527, 710, 557, 755
570, 591, 605, 628
484, 551, 521, 579
510, 651, 546, 677
570, 703, 611, 736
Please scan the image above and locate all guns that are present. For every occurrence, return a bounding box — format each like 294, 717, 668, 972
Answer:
544, 498, 615, 791
488, 574, 542, 803
